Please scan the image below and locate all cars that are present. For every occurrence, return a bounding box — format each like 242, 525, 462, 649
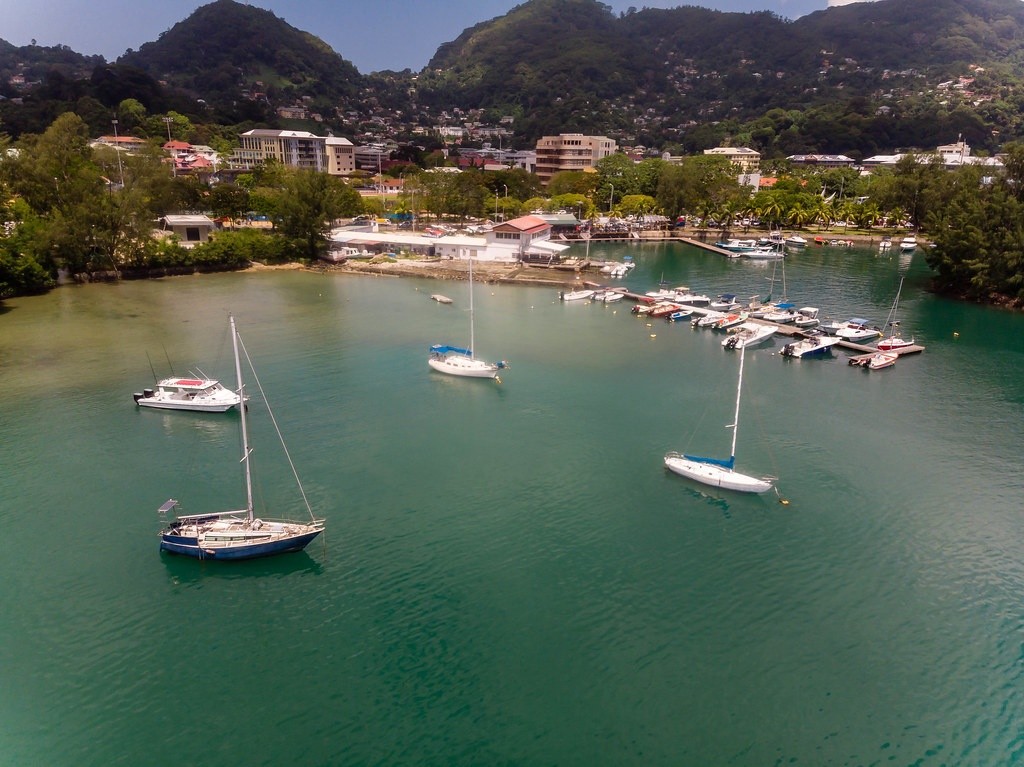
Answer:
422, 222, 492, 239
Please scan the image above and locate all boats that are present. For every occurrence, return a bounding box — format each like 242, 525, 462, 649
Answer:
869, 351, 898, 370
559, 226, 878, 344
899, 232, 919, 251
777, 335, 841, 358
879, 239, 892, 248
134, 347, 249, 412
721, 322, 779, 350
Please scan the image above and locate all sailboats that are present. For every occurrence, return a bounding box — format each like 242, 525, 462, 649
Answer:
427, 259, 510, 378
662, 340, 780, 493
877, 277, 916, 350
147, 313, 326, 562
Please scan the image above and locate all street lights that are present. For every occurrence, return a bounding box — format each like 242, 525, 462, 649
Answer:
502, 184, 508, 222
609, 183, 614, 213
